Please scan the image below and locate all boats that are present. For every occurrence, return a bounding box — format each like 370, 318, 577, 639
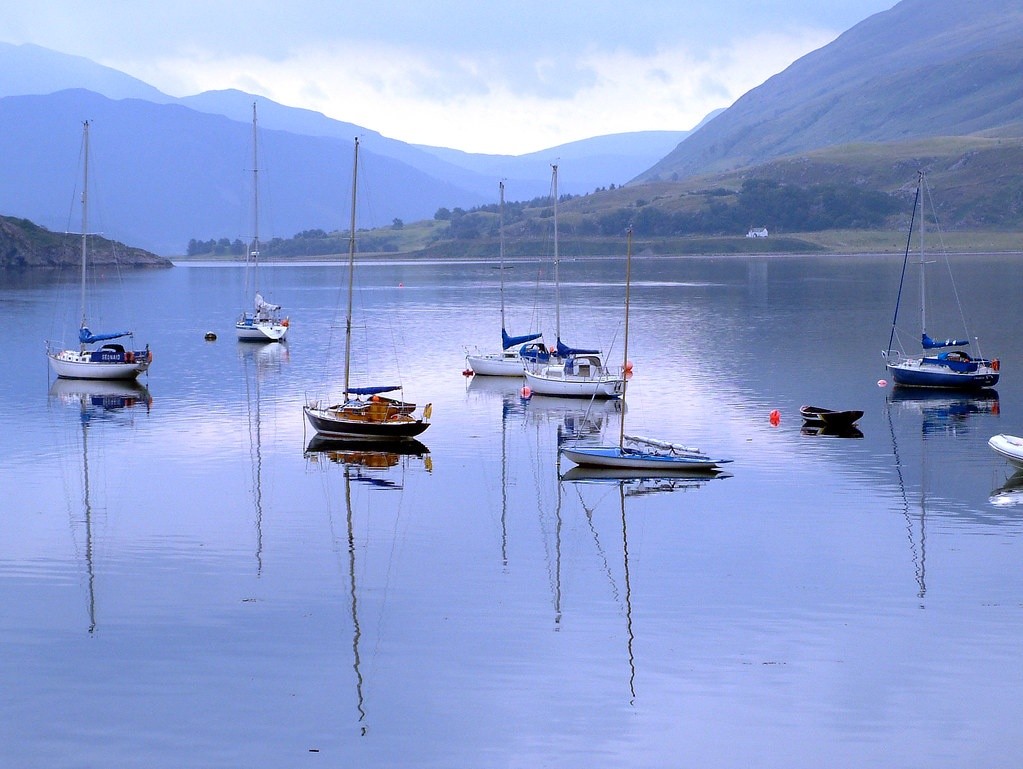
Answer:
988, 434, 1023, 471
800, 421, 865, 439
799, 405, 865, 426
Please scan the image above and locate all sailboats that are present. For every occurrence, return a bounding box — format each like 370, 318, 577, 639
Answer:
299, 133, 434, 436
235, 99, 291, 340
46, 119, 152, 380
520, 395, 626, 633
46, 374, 153, 637
302, 433, 434, 742
884, 167, 1002, 390
556, 224, 737, 470
557, 466, 736, 703
461, 178, 573, 377
236, 341, 291, 577
521, 163, 630, 399
464, 374, 552, 578
886, 386, 1001, 612
